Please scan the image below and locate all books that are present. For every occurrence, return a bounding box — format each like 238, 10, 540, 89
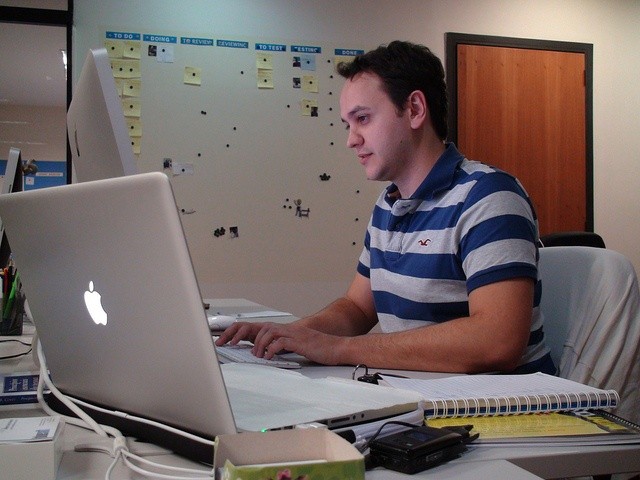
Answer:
376, 371, 620, 420
0, 368, 51, 404
424, 409, 639, 446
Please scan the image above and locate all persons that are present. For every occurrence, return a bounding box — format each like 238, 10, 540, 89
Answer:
214, 39, 558, 377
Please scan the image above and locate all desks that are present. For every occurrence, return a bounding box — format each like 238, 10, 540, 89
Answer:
2, 292, 634, 479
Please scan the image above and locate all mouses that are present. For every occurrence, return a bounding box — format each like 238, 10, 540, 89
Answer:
206, 313, 237, 332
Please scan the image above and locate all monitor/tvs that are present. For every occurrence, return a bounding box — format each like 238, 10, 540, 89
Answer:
0, 146, 23, 275
64, 49, 139, 183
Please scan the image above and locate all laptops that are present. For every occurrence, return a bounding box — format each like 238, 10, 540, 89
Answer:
0, 174, 425, 441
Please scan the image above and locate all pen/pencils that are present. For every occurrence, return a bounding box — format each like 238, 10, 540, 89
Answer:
0, 265, 22, 334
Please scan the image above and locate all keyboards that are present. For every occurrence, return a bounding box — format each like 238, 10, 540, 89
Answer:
211, 341, 301, 374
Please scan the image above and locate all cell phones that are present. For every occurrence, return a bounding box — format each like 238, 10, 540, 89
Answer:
370, 424, 466, 475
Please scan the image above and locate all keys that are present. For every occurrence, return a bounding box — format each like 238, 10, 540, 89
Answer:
359, 373, 383, 384
